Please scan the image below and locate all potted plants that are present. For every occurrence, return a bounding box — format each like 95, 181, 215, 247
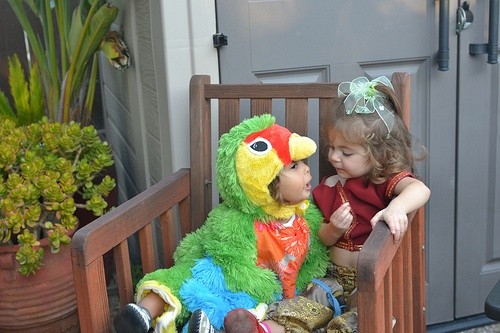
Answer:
0, 116, 116, 332
0, 0, 131, 288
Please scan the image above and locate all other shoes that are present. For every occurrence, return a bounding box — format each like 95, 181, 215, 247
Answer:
190, 309, 215, 333
113, 303, 152, 333
224, 309, 265, 333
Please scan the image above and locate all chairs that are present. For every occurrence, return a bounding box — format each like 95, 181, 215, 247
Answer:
69, 73, 424, 332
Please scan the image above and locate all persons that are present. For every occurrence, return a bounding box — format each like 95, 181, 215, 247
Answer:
113, 114, 324, 333
223, 76, 431, 333
